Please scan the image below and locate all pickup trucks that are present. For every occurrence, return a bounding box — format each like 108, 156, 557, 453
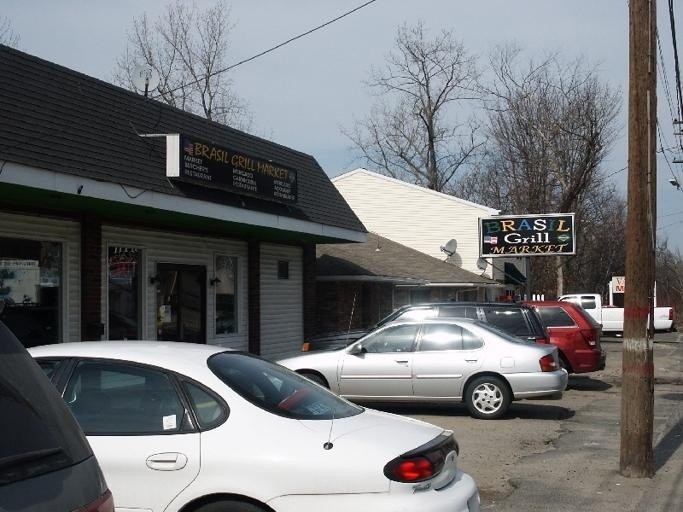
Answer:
555, 292, 674, 338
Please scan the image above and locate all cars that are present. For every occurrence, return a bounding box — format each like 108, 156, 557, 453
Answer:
262, 316, 569, 420
